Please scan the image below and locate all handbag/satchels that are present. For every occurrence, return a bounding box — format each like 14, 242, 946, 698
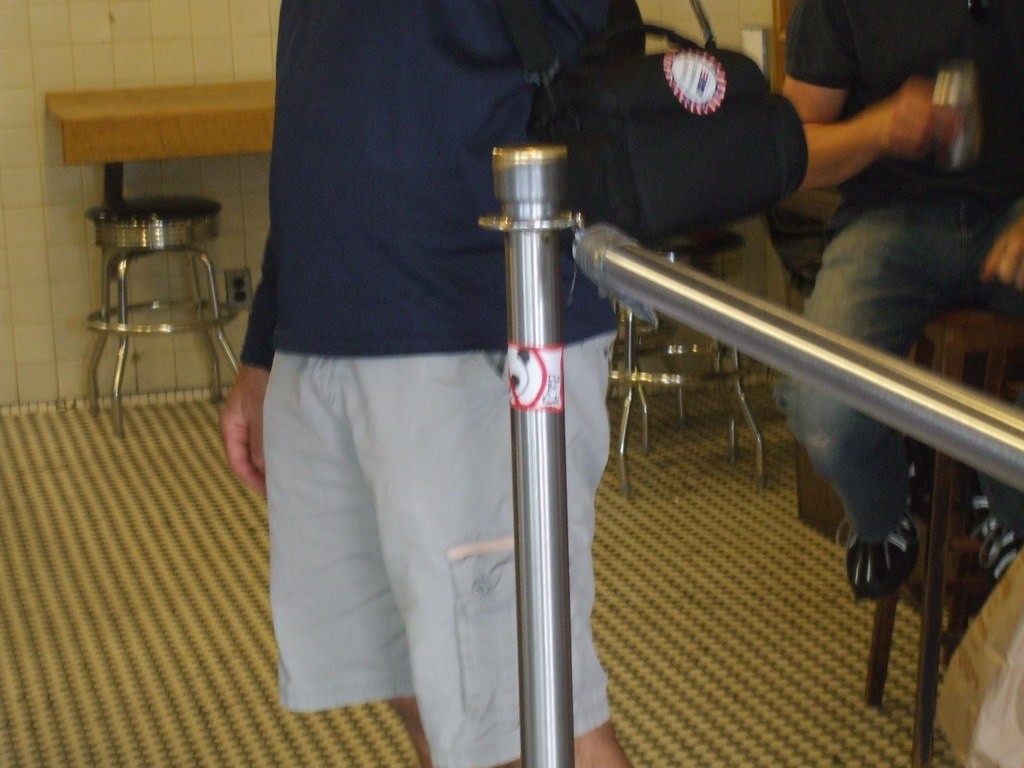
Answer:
543, 41, 809, 247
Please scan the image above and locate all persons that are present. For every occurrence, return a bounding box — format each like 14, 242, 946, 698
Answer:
781, 1, 1023, 603
220, 0, 708, 768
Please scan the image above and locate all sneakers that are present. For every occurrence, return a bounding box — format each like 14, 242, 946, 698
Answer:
836, 453, 922, 599
970, 486, 1019, 589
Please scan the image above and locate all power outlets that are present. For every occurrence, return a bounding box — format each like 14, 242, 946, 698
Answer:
224, 269, 253, 311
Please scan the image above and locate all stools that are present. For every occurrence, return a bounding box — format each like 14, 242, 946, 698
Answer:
863, 311, 1024, 768
83, 195, 241, 437
606, 227, 764, 498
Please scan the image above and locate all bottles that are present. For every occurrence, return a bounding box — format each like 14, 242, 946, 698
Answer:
928, 52, 984, 175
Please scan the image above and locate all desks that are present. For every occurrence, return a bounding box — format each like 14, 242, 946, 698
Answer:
44, 80, 276, 203
